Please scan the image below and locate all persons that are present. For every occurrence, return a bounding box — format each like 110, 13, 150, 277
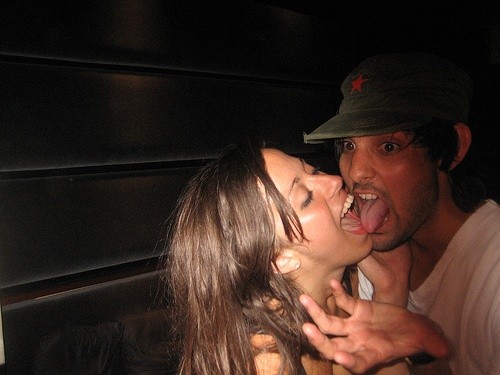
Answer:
301, 47, 499, 373
162, 145, 414, 374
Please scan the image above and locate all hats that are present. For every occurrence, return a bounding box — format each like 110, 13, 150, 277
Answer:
304, 54, 475, 142
